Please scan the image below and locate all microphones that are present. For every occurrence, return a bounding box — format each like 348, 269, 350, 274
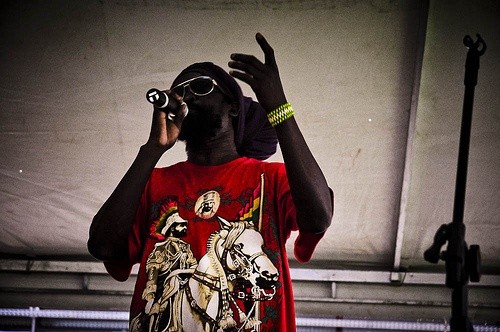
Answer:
146, 88, 188, 118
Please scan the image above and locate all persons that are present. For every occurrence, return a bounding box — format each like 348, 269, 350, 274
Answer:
86, 31, 334, 332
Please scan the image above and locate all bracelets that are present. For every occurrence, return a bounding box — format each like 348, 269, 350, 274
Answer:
267, 102, 296, 127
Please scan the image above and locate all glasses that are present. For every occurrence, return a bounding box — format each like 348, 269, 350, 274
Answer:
169, 76, 226, 100
